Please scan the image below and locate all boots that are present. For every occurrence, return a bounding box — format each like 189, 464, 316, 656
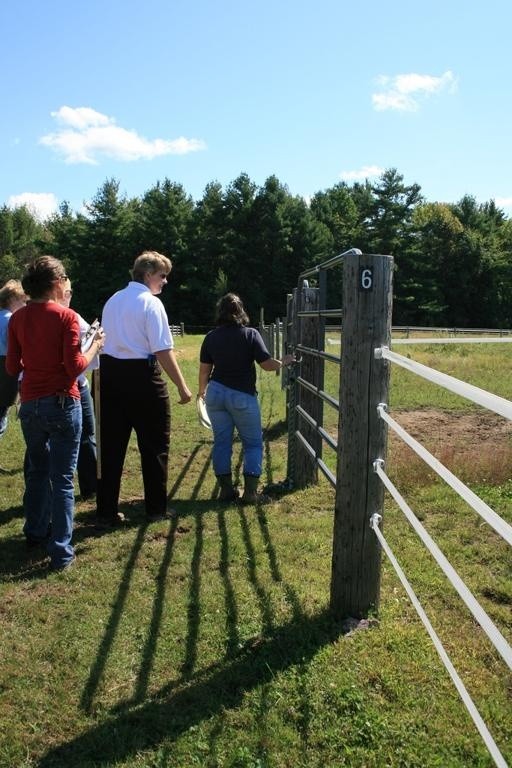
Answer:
216, 473, 240, 502
242, 475, 265, 504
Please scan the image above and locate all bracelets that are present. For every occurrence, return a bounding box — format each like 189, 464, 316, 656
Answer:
197, 392, 206, 396
93, 341, 101, 354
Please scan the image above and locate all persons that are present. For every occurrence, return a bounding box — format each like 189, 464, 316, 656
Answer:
0, 277, 27, 435
6, 253, 105, 572
57, 278, 100, 499
95, 249, 193, 530
197, 292, 297, 506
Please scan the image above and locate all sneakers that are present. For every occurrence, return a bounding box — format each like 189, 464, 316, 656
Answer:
146, 512, 178, 522
95, 512, 128, 529
